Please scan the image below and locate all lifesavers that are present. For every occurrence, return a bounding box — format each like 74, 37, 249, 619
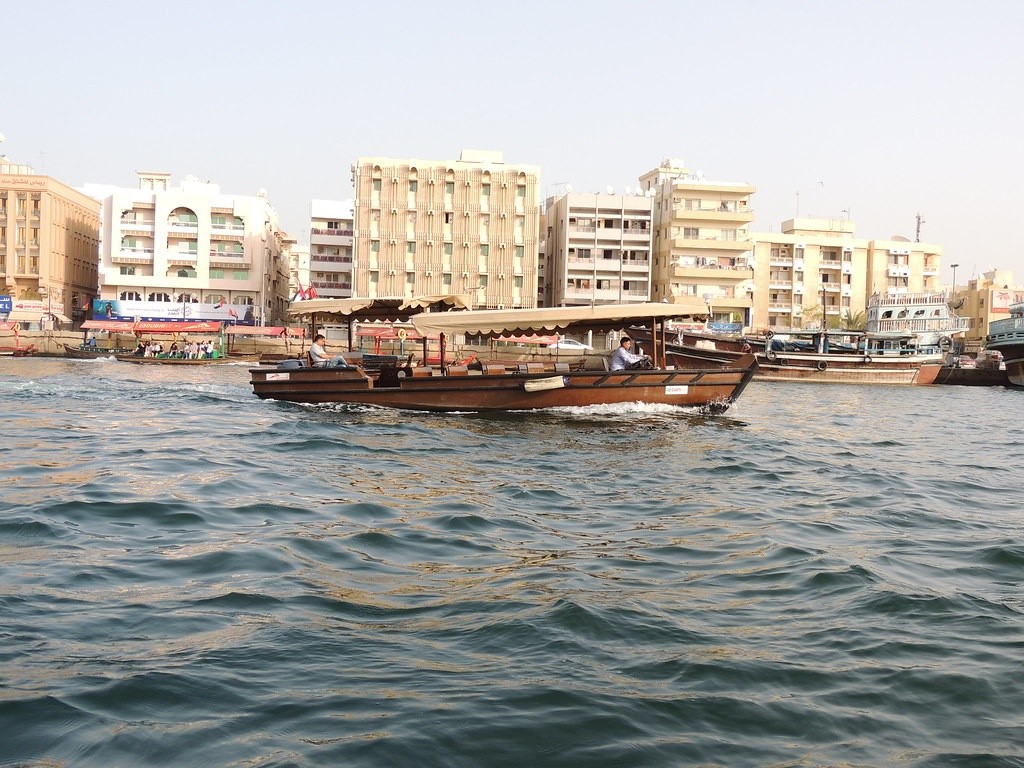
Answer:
780, 358, 790, 366
765, 331, 774, 340
740, 339, 747, 345
818, 360, 828, 371
863, 356, 872, 365
767, 350, 777, 361
14, 323, 21, 332
938, 336, 953, 351
398, 329, 406, 340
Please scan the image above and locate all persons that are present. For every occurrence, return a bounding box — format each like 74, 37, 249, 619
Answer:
609, 337, 652, 371
89, 336, 97, 347
310, 334, 347, 367
134, 340, 213, 359
105, 336, 111, 347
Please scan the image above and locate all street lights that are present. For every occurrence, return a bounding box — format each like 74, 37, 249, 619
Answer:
174, 291, 197, 320
37, 282, 62, 330
951, 263, 960, 328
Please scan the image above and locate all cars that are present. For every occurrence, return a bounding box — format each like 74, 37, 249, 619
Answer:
546, 339, 594, 350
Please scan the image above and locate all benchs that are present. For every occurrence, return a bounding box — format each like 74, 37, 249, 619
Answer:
80, 345, 135, 353
155, 349, 219, 359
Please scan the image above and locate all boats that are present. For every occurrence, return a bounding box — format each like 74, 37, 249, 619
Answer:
224, 325, 308, 358
248, 293, 760, 413
621, 284, 954, 386
985, 301, 1024, 388
63, 320, 240, 365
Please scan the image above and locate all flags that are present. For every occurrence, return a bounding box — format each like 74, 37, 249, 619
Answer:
298, 283, 306, 299
309, 282, 316, 298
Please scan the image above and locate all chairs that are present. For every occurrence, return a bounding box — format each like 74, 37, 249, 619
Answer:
602, 358, 610, 372
445, 366, 469, 375
517, 364, 528, 373
482, 365, 505, 375
412, 366, 432, 378
305, 350, 335, 367
553, 363, 570, 372
526, 362, 544, 373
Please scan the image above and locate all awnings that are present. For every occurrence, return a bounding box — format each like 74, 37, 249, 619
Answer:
53, 313, 73, 324
